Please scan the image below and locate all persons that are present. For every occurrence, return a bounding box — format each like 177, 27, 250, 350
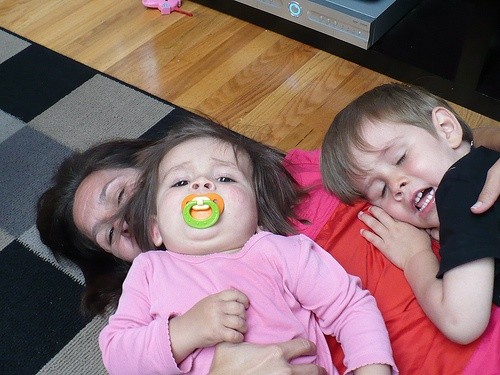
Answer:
98, 116, 400, 375
36, 134, 500, 375
321, 81, 499, 346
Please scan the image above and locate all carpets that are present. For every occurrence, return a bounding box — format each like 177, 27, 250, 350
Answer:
0, 27, 287, 375
